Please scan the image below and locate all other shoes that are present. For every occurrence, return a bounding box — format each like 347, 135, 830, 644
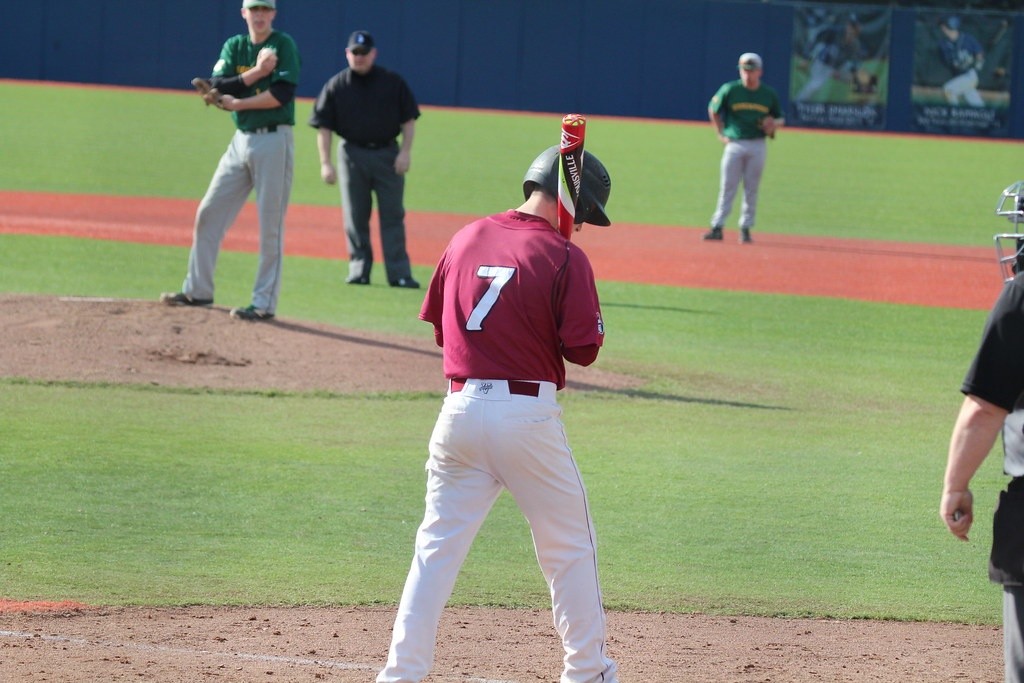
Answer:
703, 227, 723, 239
345, 275, 369, 284
739, 227, 750, 241
391, 276, 418, 288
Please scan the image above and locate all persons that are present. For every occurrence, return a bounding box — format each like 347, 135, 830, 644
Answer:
939, 181, 1022, 682
703, 52, 785, 244
797, 12, 865, 102
159, 0, 301, 322
372, 145, 616, 683
309, 31, 424, 288
936, 14, 988, 108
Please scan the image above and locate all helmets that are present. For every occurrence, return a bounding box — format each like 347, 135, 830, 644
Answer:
523, 144, 611, 226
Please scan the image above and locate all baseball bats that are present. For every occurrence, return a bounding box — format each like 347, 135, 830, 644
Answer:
557, 112, 587, 238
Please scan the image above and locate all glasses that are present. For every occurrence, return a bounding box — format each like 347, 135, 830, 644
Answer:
351, 48, 371, 55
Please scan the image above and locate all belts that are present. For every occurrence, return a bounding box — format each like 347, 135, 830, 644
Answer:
451, 378, 540, 396
246, 125, 278, 133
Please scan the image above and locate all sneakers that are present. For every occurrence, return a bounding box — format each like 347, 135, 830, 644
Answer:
159, 290, 213, 305
231, 306, 273, 320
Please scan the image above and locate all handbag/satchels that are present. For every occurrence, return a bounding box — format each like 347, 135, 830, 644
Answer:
987, 478, 1024, 595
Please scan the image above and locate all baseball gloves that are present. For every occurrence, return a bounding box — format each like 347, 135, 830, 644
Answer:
190, 76, 229, 112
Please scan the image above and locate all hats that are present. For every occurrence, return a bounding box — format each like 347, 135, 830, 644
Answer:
242, 0, 275, 10
347, 31, 374, 48
738, 54, 762, 70
